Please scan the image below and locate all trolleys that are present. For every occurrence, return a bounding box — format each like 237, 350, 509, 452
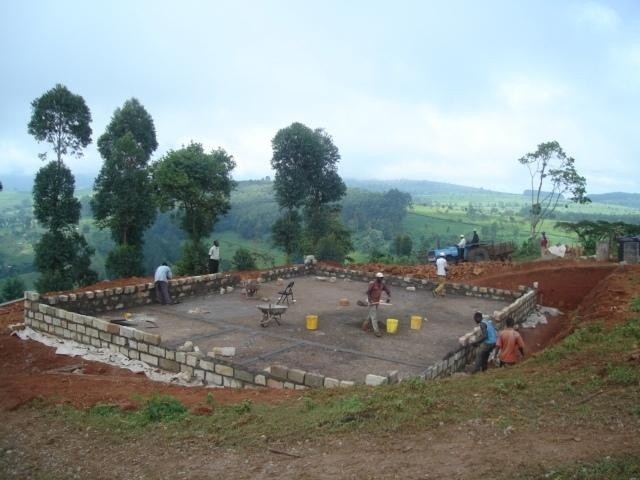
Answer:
256, 302, 289, 328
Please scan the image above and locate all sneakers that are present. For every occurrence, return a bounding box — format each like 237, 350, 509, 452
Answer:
375, 332, 381, 336
439, 294, 446, 297
432, 290, 437, 297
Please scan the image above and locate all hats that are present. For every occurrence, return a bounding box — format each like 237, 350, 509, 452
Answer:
440, 252, 445, 256
460, 235, 464, 238
376, 272, 383, 278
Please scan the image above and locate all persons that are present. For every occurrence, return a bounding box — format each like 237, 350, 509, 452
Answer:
471, 229, 479, 248
432, 252, 451, 298
153, 262, 175, 304
493, 316, 526, 369
455, 234, 467, 259
208, 240, 221, 273
460, 310, 496, 375
538, 230, 548, 257
360, 272, 390, 337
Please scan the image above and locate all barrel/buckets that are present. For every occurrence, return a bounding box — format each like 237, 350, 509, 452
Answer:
305, 314, 318, 330
623, 241, 639, 264
386, 318, 398, 333
595, 239, 610, 262
411, 315, 422, 330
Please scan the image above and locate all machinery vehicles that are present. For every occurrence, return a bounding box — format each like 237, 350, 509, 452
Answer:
426, 236, 490, 263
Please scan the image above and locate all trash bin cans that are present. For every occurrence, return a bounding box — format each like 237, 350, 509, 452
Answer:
617, 237, 640, 264
595, 241, 609, 262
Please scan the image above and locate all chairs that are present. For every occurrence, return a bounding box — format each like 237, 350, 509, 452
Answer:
277, 281, 294, 305
